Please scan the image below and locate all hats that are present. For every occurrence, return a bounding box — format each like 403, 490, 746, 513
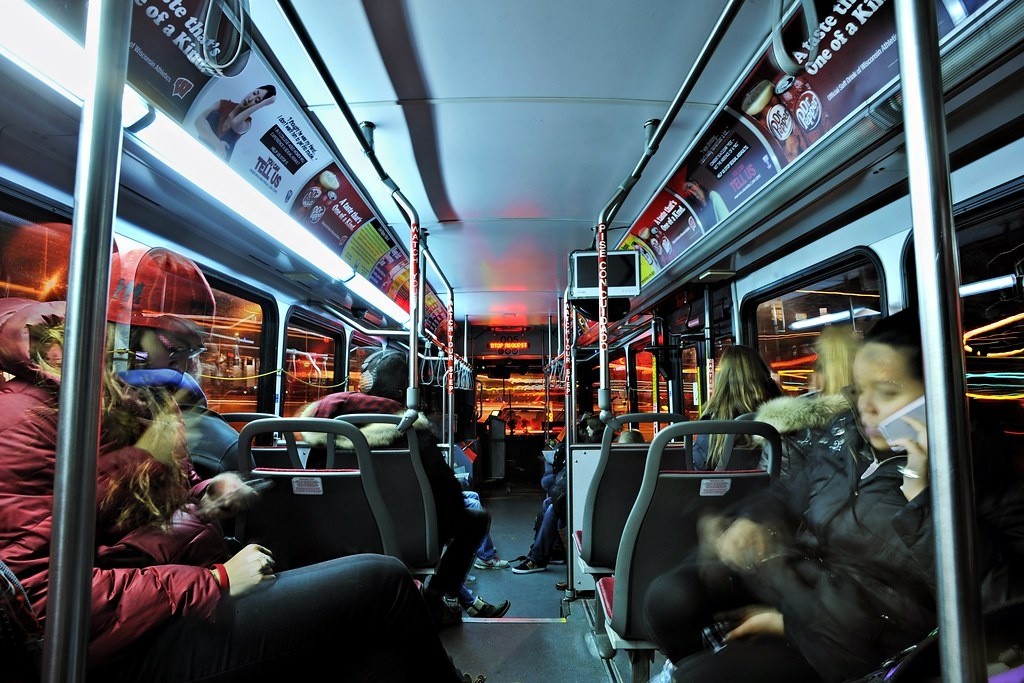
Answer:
102, 245, 220, 335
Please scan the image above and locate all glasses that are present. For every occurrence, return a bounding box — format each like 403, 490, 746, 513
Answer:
154, 328, 202, 362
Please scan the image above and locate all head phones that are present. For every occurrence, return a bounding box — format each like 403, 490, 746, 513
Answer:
357, 348, 404, 395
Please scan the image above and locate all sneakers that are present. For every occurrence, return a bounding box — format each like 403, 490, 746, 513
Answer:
649, 657, 678, 683
424, 594, 464, 627
474, 556, 510, 570
511, 559, 546, 574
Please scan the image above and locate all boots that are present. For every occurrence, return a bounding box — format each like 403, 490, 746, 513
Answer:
556, 526, 568, 590
466, 596, 511, 618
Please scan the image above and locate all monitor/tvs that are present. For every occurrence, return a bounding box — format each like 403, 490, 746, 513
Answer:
571, 251, 641, 298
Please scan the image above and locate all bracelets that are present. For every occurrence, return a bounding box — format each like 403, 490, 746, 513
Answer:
212, 562, 229, 598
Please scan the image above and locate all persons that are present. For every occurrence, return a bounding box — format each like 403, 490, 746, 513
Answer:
514, 415, 645, 574
293, 349, 512, 617
0, 248, 472, 683
642, 305, 936, 682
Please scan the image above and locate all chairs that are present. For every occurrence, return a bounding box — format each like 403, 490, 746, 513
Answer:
184, 413, 451, 591
547, 408, 782, 683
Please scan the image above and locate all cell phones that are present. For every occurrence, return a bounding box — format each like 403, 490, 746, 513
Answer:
877, 395, 928, 453
